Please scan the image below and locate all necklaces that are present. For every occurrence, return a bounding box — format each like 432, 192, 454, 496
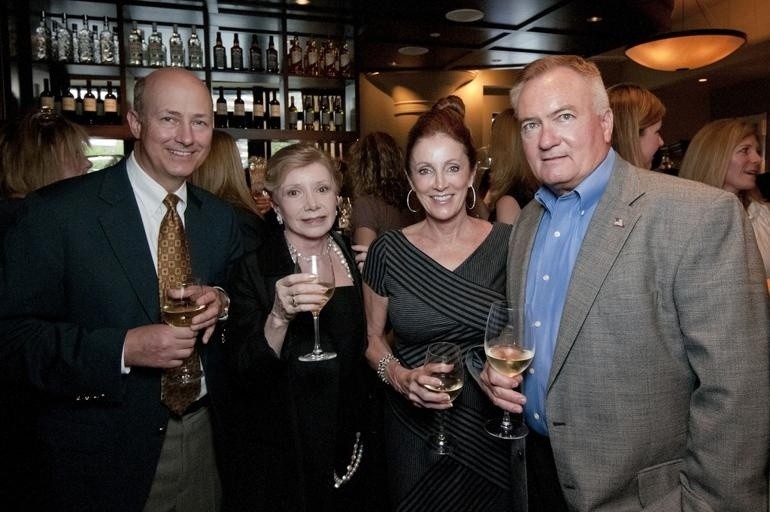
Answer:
283, 231, 355, 287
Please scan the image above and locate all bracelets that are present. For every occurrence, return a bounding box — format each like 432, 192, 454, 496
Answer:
375, 354, 399, 389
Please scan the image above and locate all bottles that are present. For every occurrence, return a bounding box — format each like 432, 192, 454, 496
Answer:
270, 90, 280, 129
75, 87, 84, 124
128, 21, 142, 66
289, 96, 297, 130
214, 32, 226, 69
112, 28, 120, 65
304, 42, 313, 74
234, 87, 245, 129
304, 98, 314, 131
319, 39, 327, 74
231, 33, 243, 70
340, 36, 353, 75
92, 25, 101, 64
58, 12, 72, 62
101, 16, 114, 65
79, 14, 94, 64
104, 81, 118, 125
334, 97, 345, 132
288, 40, 295, 73
189, 25, 202, 68
61, 82, 75, 121
325, 33, 335, 75
254, 90, 263, 129
72, 24, 80, 63
40, 79, 54, 109
307, 33, 319, 77
140, 30, 149, 66
36, 9, 51, 60
250, 35, 262, 71
320, 99, 330, 131
96, 87, 104, 124
48, 21, 59, 61
149, 23, 162, 67
291, 34, 302, 75
267, 37, 277, 73
169, 23, 184, 68
217, 86, 227, 127
84, 80, 96, 126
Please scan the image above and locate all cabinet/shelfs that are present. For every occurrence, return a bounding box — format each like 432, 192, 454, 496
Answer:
0, 0, 360, 167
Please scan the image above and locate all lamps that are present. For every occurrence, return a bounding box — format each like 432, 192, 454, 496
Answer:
622, 30, 748, 78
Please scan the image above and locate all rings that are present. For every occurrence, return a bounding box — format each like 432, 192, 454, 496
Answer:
291, 297, 296, 307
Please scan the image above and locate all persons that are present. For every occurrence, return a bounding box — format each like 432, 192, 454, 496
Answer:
361, 95, 536, 511
677, 113, 770, 283
484, 106, 538, 227
606, 79, 668, 171
185, 127, 264, 221
345, 128, 426, 255
0, 67, 275, 510
484, 54, 770, 510
215, 141, 365, 510
1, 111, 95, 200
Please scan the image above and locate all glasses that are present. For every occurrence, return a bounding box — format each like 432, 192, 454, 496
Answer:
35, 103, 54, 152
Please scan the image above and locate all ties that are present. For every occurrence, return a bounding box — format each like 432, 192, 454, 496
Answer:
157, 192, 202, 415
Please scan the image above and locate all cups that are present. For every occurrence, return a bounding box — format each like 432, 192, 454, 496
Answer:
293, 253, 337, 362
161, 277, 206, 384
422, 342, 465, 455
484, 301, 536, 440
